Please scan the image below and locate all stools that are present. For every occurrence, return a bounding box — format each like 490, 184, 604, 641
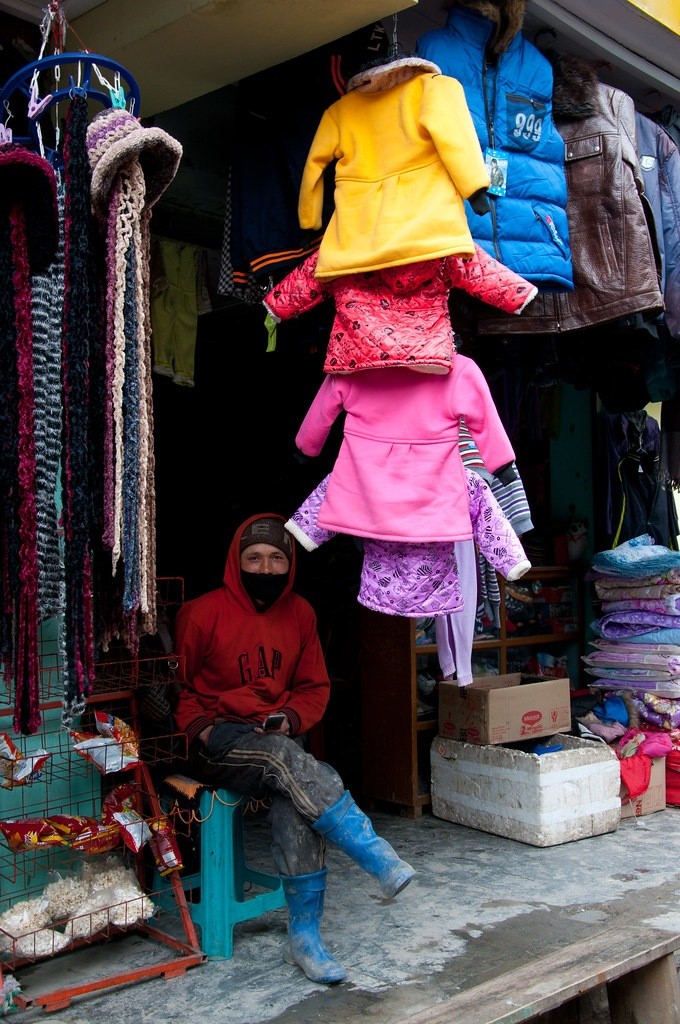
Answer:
148, 773, 289, 961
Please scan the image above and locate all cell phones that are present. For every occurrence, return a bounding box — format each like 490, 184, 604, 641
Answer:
264, 715, 285, 732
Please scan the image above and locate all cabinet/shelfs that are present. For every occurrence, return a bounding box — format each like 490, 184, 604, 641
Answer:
366, 559, 587, 818
0, 689, 208, 1013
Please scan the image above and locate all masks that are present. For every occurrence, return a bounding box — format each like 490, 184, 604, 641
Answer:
240, 568, 290, 612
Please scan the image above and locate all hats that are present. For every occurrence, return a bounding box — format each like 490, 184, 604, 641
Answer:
240, 518, 292, 562
0, 143, 60, 276
86, 107, 183, 223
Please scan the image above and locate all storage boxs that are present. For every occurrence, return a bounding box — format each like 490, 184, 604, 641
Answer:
665, 743, 680, 805
608, 744, 665, 820
431, 733, 622, 847
437, 672, 572, 746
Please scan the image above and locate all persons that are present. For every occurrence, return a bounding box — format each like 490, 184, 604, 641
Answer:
173, 513, 416, 983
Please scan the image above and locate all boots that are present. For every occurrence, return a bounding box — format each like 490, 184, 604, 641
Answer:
279, 866, 347, 984
310, 788, 416, 898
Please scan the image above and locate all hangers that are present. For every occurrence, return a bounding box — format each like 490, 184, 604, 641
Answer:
644, 89, 663, 114
634, 434, 648, 455
386, 42, 403, 57
